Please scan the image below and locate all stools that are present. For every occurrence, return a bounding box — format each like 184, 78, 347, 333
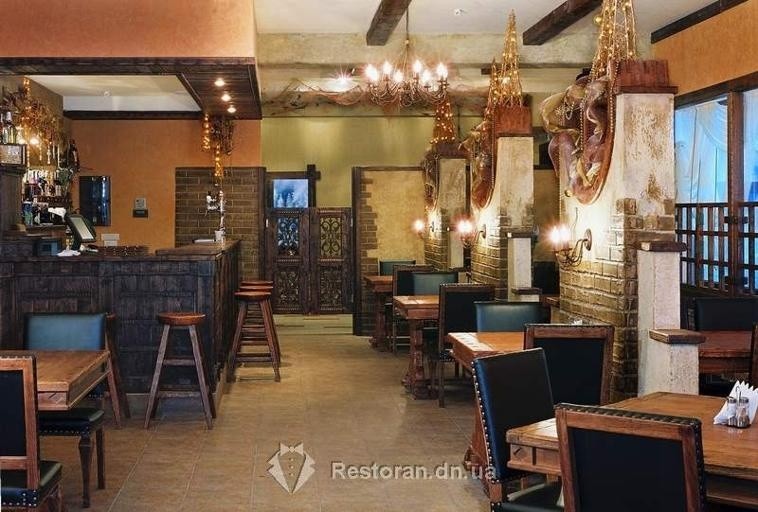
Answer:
225, 280, 281, 382
141, 309, 216, 430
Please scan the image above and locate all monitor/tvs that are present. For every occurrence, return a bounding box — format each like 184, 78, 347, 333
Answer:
65, 214, 96, 250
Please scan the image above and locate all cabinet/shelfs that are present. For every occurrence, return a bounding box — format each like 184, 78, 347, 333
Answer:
18, 167, 72, 251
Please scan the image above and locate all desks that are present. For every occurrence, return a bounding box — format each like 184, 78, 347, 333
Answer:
692, 329, 758, 373
394, 295, 440, 398
448, 330, 528, 497
504, 391, 758, 509
364, 275, 394, 348
1, 345, 111, 410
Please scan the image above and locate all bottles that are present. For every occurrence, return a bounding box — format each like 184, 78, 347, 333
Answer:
0, 112, 9, 144
70, 139, 78, 167
726, 396, 738, 426
32, 195, 41, 227
5, 110, 15, 143
736, 397, 750, 428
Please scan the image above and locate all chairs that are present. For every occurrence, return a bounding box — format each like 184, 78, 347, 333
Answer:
553, 402, 709, 512
412, 273, 461, 394
17, 310, 112, 506
474, 300, 551, 333
382, 264, 434, 350
428, 283, 497, 408
470, 344, 572, 512
521, 324, 615, 407
1, 353, 67, 510
378, 259, 415, 275
692, 292, 757, 334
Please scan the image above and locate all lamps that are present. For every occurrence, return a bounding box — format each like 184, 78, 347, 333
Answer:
549, 224, 592, 269
363, 1, 450, 109
455, 219, 486, 250
414, 219, 435, 232
0, 75, 58, 168
